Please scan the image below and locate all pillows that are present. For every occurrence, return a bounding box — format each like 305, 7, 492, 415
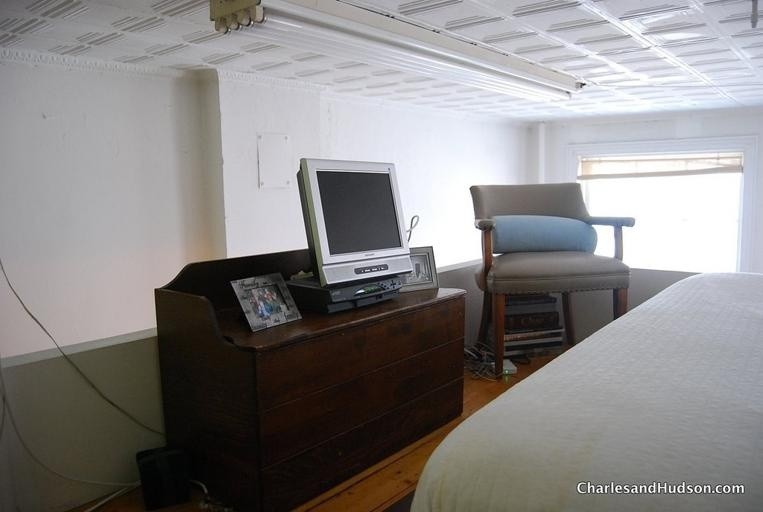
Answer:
491, 215, 598, 254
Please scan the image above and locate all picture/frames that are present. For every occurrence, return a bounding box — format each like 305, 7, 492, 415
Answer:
396, 246, 439, 293
231, 272, 303, 333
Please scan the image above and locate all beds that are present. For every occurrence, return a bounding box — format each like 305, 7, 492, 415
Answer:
411, 271, 762, 512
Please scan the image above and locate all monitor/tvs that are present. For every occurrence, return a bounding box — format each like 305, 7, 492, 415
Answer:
295, 158, 414, 286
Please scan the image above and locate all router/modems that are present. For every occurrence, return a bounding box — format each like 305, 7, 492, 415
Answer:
490, 359, 518, 375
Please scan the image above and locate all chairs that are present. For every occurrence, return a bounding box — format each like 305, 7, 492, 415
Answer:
469, 183, 635, 380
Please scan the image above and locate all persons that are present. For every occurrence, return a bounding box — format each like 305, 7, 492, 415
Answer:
250, 291, 282, 320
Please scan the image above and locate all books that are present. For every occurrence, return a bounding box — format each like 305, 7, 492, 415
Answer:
485, 294, 565, 357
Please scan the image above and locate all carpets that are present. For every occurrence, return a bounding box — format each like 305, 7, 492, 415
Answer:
372, 479, 419, 512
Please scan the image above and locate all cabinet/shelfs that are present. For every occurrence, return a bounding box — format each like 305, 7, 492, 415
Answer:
154, 249, 466, 512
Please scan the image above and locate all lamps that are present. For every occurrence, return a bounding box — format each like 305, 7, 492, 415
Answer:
208, 0, 585, 103
